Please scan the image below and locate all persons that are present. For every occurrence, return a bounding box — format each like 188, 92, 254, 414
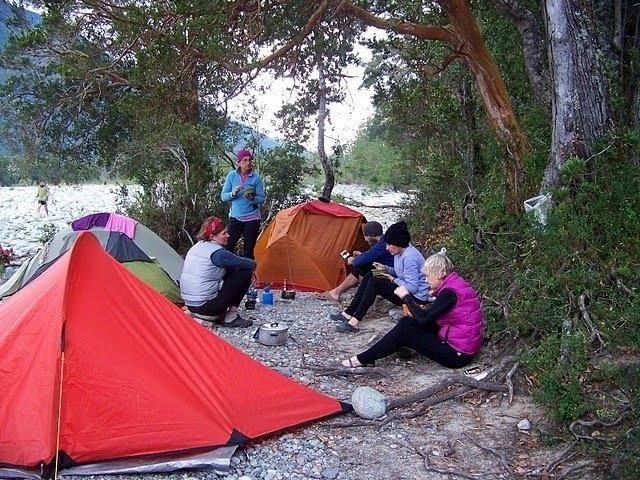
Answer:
332, 252, 484, 368
328, 221, 431, 333
34, 182, 49, 215
179, 218, 257, 329
222, 150, 267, 259
313, 221, 395, 301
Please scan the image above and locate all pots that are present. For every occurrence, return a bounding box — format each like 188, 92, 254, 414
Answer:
252, 322, 290, 346
281, 290, 296, 300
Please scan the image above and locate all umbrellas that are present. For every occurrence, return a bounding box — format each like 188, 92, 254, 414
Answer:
337, 359, 367, 368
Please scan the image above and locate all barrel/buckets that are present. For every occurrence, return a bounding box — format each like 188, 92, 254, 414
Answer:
222, 314, 253, 327
337, 321, 359, 332
331, 312, 350, 322
315, 290, 341, 303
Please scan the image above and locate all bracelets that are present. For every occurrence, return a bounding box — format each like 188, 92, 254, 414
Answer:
243, 186, 256, 195
372, 268, 388, 278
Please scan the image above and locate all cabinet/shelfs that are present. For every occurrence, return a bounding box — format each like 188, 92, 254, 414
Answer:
245, 287, 257, 310
262, 286, 274, 304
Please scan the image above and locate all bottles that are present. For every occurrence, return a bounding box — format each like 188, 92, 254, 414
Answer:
384, 221, 410, 248
364, 221, 383, 236
237, 150, 252, 163
